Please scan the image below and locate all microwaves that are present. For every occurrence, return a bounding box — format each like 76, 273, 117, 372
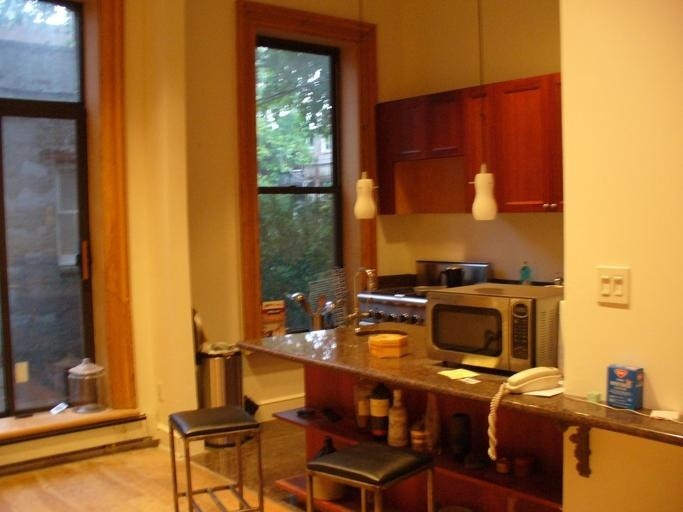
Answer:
423, 281, 564, 374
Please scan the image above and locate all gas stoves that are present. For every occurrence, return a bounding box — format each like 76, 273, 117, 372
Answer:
370, 284, 427, 300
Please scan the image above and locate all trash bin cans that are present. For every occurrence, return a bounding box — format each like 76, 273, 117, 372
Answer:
200, 342, 242, 448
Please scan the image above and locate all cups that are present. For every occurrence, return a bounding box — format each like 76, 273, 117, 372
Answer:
436, 265, 464, 288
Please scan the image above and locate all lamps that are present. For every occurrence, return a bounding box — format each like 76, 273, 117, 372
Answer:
468, 12, 499, 222
353, 7, 379, 220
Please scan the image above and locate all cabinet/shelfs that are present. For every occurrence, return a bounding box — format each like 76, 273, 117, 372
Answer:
267, 363, 683, 512
379, 79, 562, 216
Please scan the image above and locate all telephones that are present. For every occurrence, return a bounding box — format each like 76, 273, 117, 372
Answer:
505, 366, 561, 393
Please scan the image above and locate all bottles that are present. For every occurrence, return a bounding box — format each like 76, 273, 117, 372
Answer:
367, 380, 391, 441
67, 357, 110, 415
518, 260, 532, 286
351, 374, 373, 433
386, 389, 410, 448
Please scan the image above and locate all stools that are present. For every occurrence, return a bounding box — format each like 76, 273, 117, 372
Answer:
164, 405, 264, 512
